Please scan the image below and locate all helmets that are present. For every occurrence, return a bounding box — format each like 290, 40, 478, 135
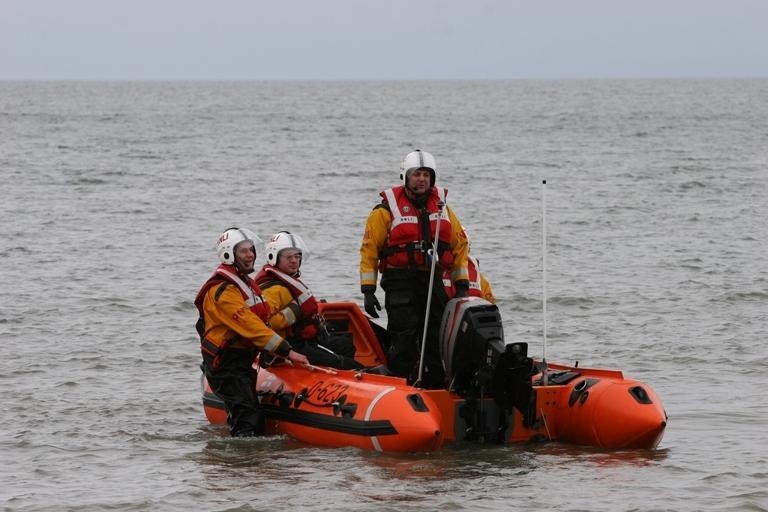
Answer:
215, 227, 264, 265
264, 230, 310, 266
399, 149, 437, 186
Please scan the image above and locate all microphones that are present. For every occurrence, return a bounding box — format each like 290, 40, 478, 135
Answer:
407, 183, 418, 190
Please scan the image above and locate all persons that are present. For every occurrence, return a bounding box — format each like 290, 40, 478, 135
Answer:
253, 229, 368, 372
358, 149, 471, 385
193, 226, 311, 439
439, 223, 499, 306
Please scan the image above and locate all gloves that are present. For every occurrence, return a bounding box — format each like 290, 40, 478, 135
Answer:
363, 290, 381, 318
455, 283, 468, 297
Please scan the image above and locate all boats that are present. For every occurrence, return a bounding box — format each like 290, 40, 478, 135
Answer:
200, 300, 669, 457
